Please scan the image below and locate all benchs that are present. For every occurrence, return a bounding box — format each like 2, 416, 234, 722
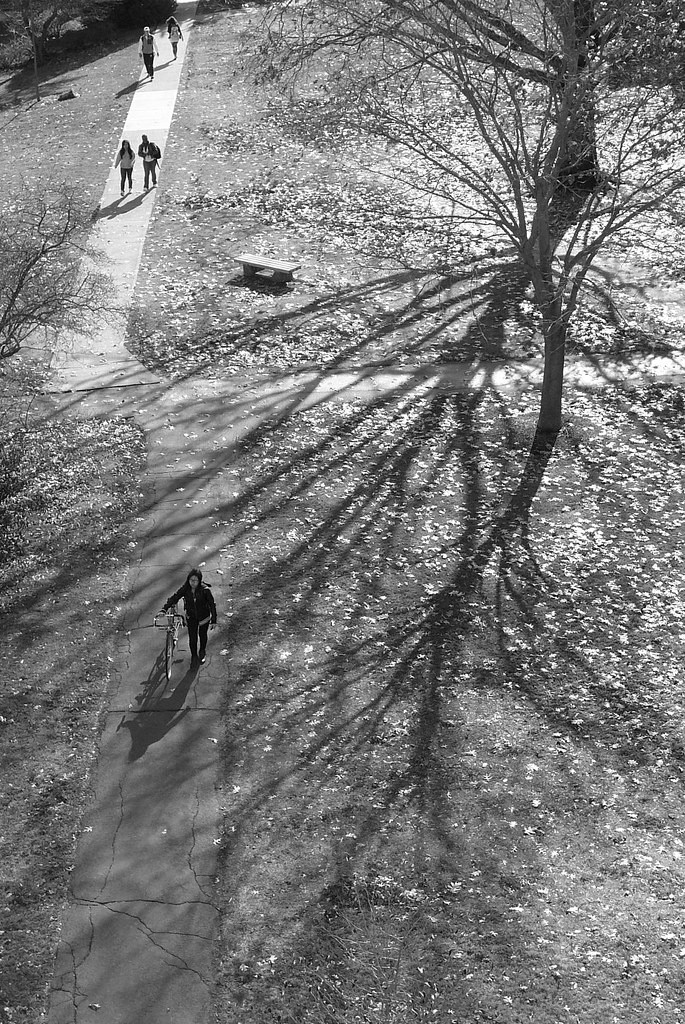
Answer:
234, 254, 302, 282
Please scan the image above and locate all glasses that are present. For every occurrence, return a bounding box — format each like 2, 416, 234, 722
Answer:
189, 579, 199, 583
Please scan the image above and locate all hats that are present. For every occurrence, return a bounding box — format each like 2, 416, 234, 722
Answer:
143, 26, 150, 31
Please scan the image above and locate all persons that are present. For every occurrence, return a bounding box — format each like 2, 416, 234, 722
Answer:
156, 569, 218, 666
138, 26, 160, 80
165, 16, 182, 59
114, 140, 136, 197
138, 135, 159, 191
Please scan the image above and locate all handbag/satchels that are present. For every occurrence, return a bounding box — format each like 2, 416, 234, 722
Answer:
149, 142, 161, 159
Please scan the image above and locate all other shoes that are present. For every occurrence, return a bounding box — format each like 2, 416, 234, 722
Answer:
147, 73, 154, 79
189, 649, 207, 671
120, 183, 158, 196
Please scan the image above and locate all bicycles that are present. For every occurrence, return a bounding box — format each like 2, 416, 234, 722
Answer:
153, 605, 187, 682
